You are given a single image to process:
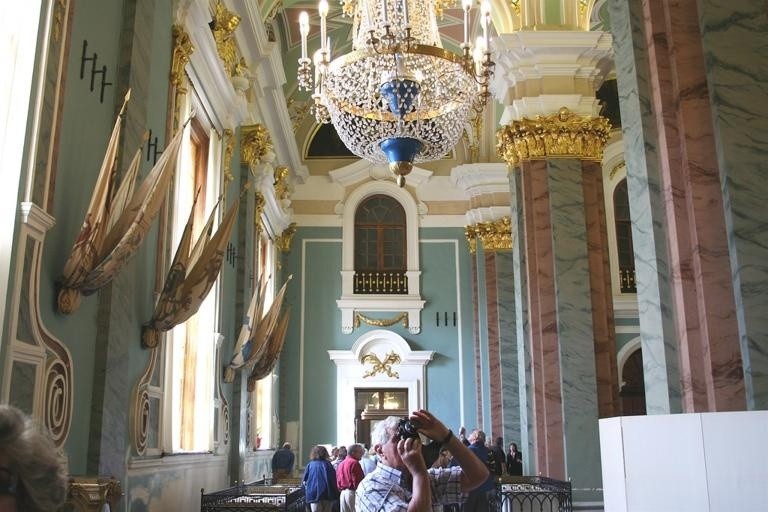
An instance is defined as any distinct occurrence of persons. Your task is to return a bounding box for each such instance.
[271,441,295,481]
[300,415,522,511]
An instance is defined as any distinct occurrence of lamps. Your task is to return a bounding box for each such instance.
[296,0,494,188]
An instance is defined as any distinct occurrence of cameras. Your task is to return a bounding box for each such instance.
[396,416,421,439]
